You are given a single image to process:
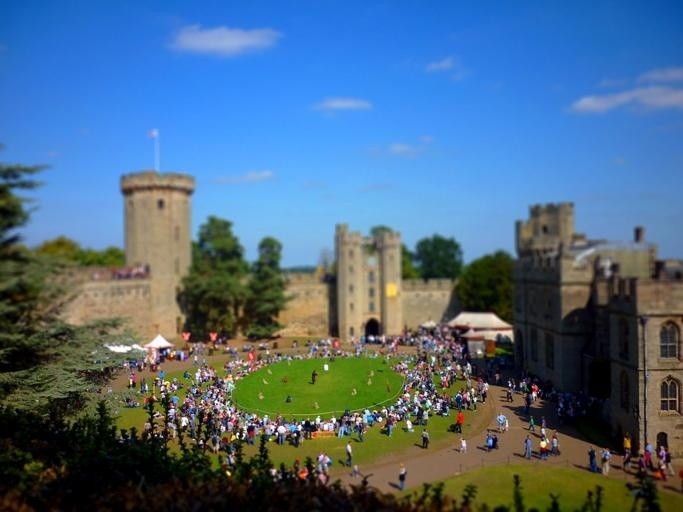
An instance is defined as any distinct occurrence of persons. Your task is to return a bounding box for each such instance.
[122,324,674,489]
